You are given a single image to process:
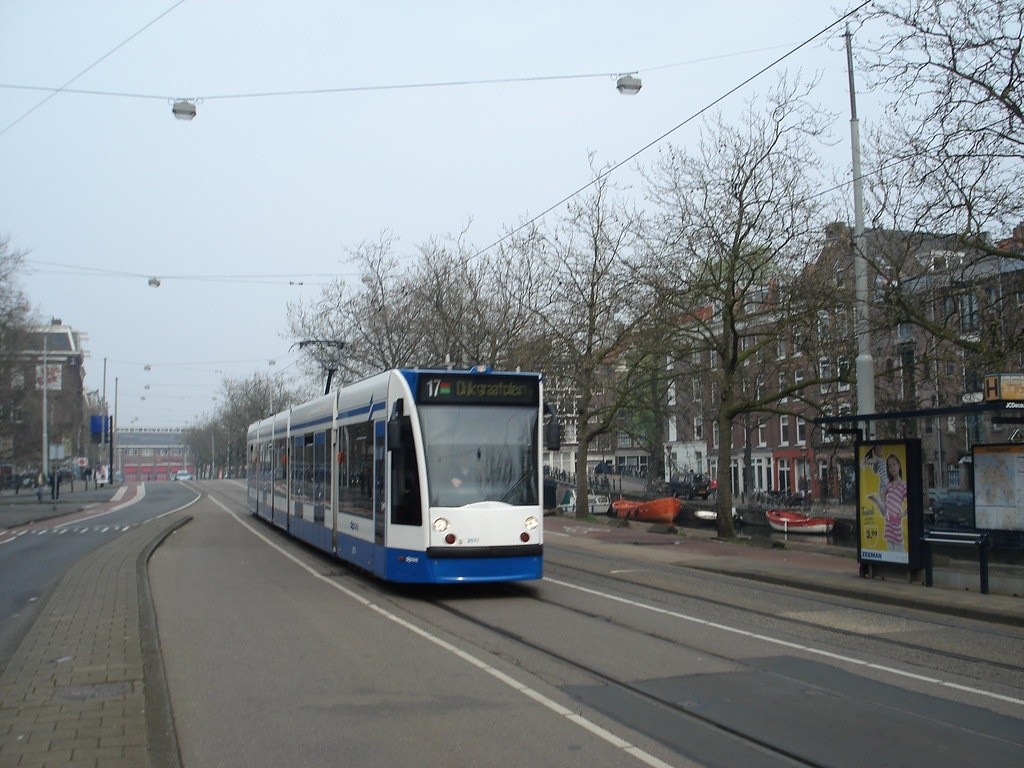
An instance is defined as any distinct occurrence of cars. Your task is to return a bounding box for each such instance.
[176,470,192,480]
[923,488,973,512]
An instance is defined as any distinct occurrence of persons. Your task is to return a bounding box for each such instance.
[867,453,907,552]
[863,446,888,497]
[49,471,61,503]
[84,466,92,479]
[444,458,481,489]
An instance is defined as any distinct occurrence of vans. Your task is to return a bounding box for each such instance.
[670,472,711,500]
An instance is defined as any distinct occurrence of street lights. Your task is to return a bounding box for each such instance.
[666,445,672,483]
[799,445,809,499]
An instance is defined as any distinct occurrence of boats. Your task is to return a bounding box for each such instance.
[766,509,834,534]
[557,488,684,523]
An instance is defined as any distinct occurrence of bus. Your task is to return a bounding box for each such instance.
[248,366,560,596]
